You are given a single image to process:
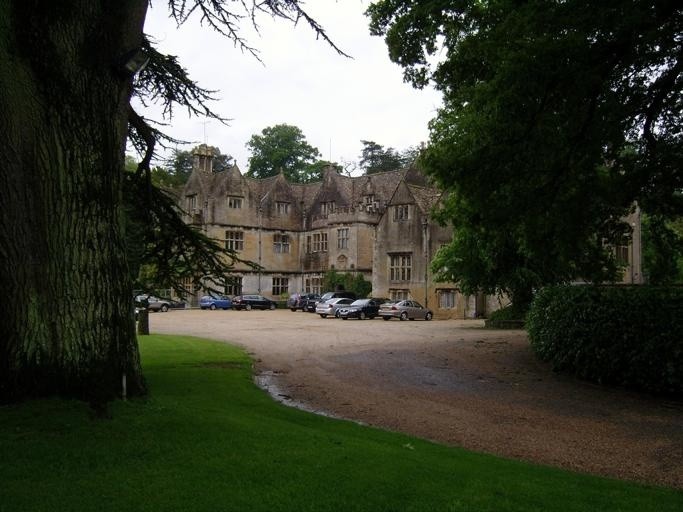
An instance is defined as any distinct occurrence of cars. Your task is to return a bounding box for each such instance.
[315,296,434,322]
[134,304,146,322]
[132,294,171,313]
[199,294,232,311]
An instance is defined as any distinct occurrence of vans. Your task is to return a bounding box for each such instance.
[230,294,278,310]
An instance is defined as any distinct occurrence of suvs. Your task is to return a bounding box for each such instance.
[304,290,358,313]
[285,292,320,313]
